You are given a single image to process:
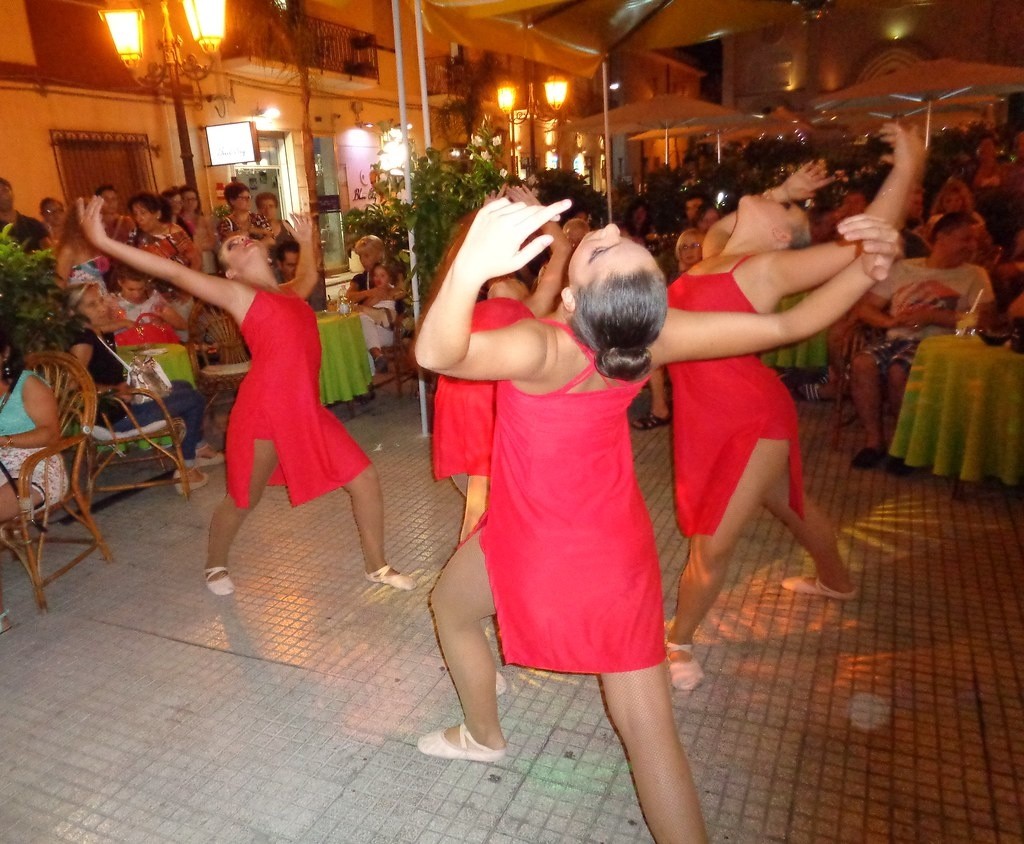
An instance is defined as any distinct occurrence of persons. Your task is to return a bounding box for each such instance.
[624,118,1024,476]
[65,278,224,493]
[0,333,69,633]
[72,194,416,595]
[419,180,570,695]
[1,172,399,460]
[416,196,904,844]
[652,120,926,687]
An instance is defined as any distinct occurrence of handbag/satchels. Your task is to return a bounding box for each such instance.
[112,312,181,347]
[122,356,172,405]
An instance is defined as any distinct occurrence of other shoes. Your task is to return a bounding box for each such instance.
[202,566,235,596]
[780,575,856,601]
[494,670,507,698]
[848,446,887,469]
[416,722,507,762]
[363,560,418,591]
[663,633,705,691]
[797,381,821,401]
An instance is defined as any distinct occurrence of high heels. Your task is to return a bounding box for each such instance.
[172,465,210,495]
[192,440,226,466]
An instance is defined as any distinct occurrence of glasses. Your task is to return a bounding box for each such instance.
[239,195,252,201]
[183,196,196,202]
[173,199,183,205]
[42,208,64,214]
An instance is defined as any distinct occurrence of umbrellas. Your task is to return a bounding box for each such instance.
[552,93,744,198]
[695,106,862,144]
[809,57,1024,151]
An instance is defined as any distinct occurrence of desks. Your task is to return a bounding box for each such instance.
[312,308,376,421]
[74,344,197,454]
[757,293,830,382]
[885,334,1024,503]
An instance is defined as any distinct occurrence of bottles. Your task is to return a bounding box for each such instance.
[338,285,350,316]
[1011,316,1024,353]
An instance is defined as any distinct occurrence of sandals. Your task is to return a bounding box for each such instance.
[0,607,12,635]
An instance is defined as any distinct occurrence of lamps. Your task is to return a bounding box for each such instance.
[352,102,375,130]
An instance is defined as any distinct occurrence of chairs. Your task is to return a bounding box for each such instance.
[0,351,115,615]
[60,358,192,506]
[359,302,418,401]
[187,300,252,454]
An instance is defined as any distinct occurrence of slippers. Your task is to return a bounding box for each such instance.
[631,409,672,429]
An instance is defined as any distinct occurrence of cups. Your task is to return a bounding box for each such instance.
[325,299,337,311]
[979,303,1010,345]
[134,322,150,350]
[955,308,979,340]
